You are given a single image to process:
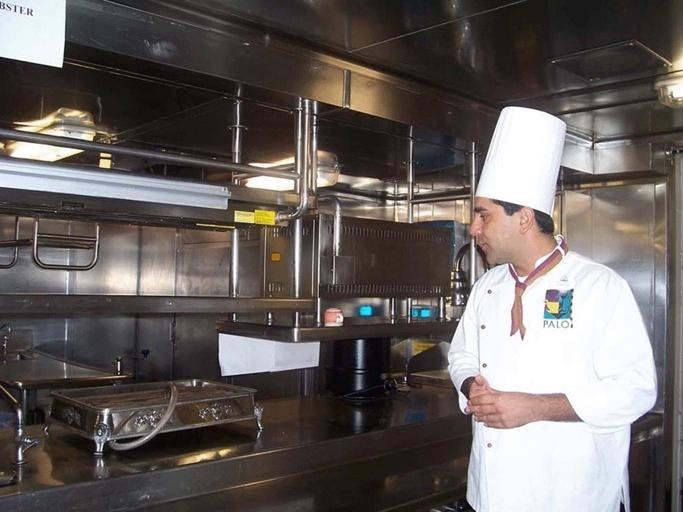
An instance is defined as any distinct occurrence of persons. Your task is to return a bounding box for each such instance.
[445,193,660,511]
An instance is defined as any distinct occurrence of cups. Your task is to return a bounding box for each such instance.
[323,307,344,327]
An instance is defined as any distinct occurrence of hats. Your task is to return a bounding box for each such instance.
[474,104,568,217]
